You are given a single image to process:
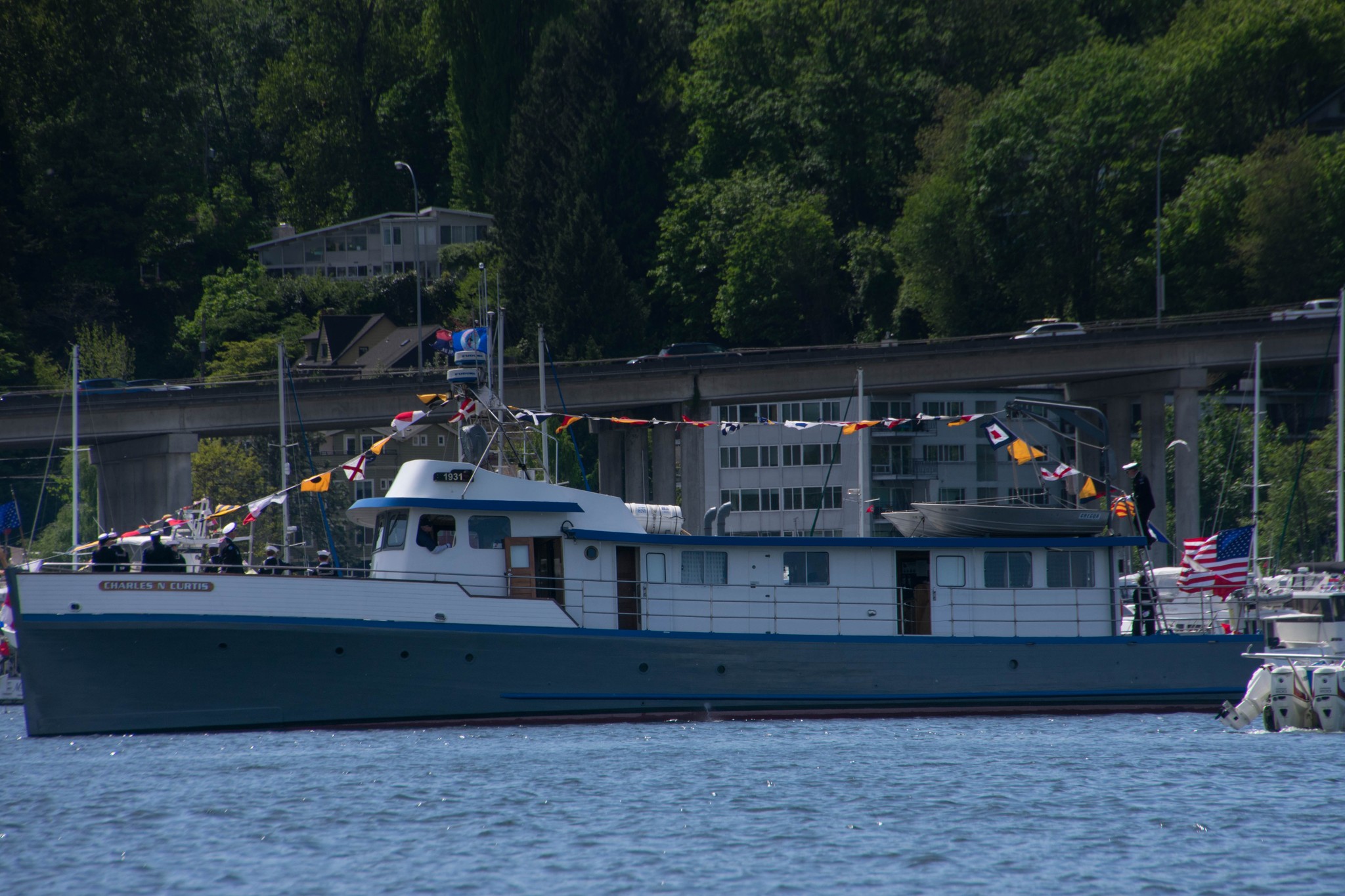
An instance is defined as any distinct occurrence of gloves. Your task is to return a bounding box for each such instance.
[1143,611,1149,618]
[446,543,451,548]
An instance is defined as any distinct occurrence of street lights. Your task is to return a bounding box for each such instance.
[1156,124,1183,331]
[393,160,424,384]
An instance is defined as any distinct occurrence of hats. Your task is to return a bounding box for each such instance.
[265,546,278,553]
[150,530,159,536]
[419,518,435,527]
[223,522,238,534]
[108,532,117,538]
[97,533,108,541]
[206,543,219,548]
[166,540,179,546]
[317,550,330,556]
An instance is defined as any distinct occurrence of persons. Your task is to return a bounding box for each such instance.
[258,546,314,575]
[1122,462,1156,549]
[311,550,339,577]
[140,531,187,574]
[204,543,222,573]
[1132,575,1158,636]
[219,522,244,574]
[416,518,451,553]
[92,533,131,574]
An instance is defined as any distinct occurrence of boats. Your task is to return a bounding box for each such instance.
[0,261,1271,739]
[1118,567,1345,735]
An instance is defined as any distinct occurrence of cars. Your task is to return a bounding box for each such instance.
[626,343,743,365]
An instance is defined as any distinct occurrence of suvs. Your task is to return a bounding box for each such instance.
[76,378,194,394]
[0,392,55,401]
[1008,323,1086,340]
[1271,300,1343,323]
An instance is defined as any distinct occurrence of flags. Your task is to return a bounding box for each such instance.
[370,393,457,458]
[1176,524,1256,602]
[0,500,21,534]
[980,416,1080,481]
[429,327,490,357]
[507,405,985,436]
[448,396,485,424]
[1079,476,1170,544]
[341,452,366,481]
[0,559,44,664]
[301,470,332,492]
[65,490,287,554]
